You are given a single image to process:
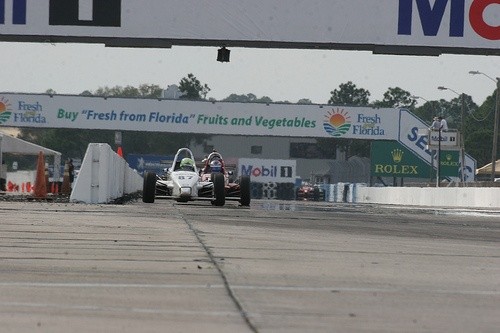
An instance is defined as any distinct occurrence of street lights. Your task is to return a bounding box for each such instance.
[409,95,433,125]
[469,70,500,183]
[437,86,465,187]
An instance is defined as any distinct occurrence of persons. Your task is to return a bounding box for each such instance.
[430,117,448,131]
[175,158,194,171]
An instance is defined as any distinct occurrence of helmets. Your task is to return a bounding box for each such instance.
[210,160,221,167]
[180,158,194,168]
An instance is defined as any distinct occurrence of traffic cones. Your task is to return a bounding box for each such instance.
[44,160,50,193]
[58,160,71,195]
[117,146,123,158]
[24,150,53,200]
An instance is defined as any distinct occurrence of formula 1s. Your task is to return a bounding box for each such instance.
[143,148,250,207]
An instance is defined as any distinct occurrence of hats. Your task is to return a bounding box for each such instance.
[433,117,439,120]
